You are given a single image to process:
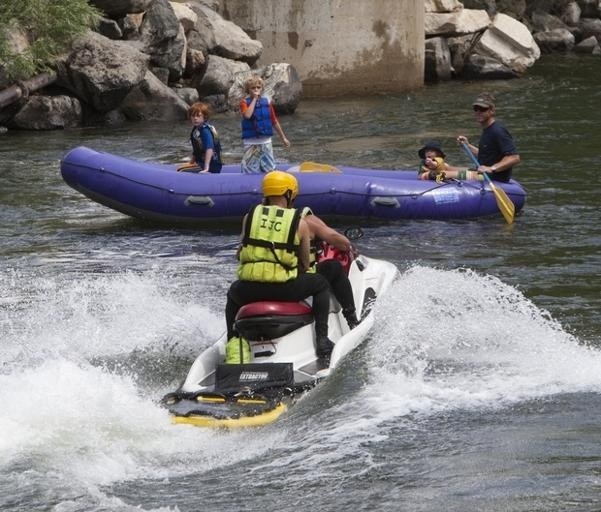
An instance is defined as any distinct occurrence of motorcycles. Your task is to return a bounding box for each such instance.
[150,224,405,432]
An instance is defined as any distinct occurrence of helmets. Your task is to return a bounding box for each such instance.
[262,170,298,198]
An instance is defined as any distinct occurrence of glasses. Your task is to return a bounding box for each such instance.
[473,105,488,112]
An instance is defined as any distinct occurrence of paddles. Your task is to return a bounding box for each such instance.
[460,139,515,225]
[299,162,342,173]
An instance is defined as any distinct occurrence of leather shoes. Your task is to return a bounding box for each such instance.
[316,336,336,355]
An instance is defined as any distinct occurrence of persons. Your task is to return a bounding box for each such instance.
[416,138,452,180]
[225,170,339,361]
[289,173,378,332]
[437,90,523,184]
[176,99,224,177]
[238,73,293,174]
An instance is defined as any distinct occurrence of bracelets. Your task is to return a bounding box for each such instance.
[491,166,498,174]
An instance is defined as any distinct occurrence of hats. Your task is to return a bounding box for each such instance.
[472,92,496,109]
[418,140,446,158]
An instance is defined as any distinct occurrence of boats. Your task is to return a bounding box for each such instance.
[56,143,529,231]
